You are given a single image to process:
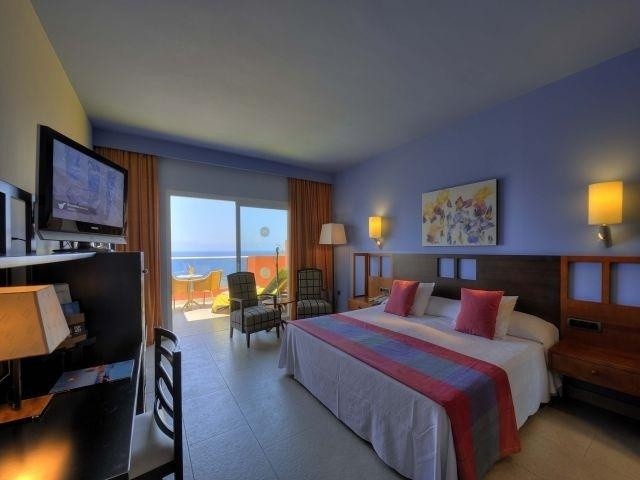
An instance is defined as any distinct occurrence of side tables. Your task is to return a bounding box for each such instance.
[262,297,297,337]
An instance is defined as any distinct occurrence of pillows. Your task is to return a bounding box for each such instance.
[454,287,503,341]
[491,295,522,339]
[383,279,418,318]
[408,281,437,318]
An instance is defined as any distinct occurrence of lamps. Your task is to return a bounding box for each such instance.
[0,285,71,424]
[368,216,384,250]
[317,222,348,313]
[586,180,623,248]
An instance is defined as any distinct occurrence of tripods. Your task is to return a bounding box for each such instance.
[266,261,288,333]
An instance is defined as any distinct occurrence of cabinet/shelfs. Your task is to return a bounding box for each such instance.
[34,250,145,415]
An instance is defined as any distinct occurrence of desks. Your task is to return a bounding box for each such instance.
[1,343,144,480]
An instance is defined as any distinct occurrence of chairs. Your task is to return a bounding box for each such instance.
[171,265,291,313]
[296,267,334,319]
[107,325,183,479]
[226,271,281,347]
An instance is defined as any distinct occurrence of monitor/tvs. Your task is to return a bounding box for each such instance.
[35,123,127,254]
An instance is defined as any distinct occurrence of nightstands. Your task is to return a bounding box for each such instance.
[347,296,382,312]
[547,338,640,420]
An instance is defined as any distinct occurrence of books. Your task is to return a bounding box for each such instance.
[48,358,135,394]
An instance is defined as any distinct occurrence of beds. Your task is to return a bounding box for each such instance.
[283,295,558,479]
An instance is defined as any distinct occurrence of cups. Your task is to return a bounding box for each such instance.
[100,243,111,250]
[36,234,53,255]
